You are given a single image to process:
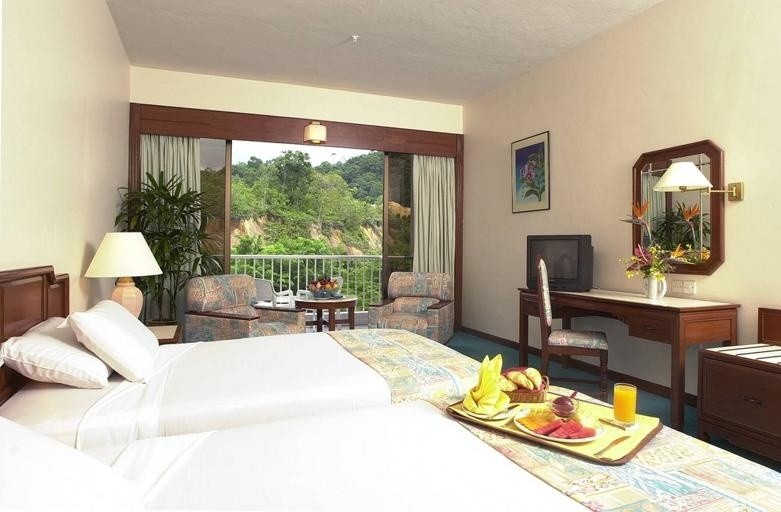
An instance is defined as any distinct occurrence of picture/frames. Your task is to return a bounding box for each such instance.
[511,131,550,213]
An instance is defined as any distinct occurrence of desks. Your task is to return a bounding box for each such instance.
[518,288,740,433]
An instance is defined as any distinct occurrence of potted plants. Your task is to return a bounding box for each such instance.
[114,170,224,343]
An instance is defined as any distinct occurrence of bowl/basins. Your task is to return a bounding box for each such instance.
[307,277,343,298]
[550,396,579,418]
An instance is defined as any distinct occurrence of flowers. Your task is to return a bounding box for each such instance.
[618,197,685,278]
[308,277,339,292]
[679,202,710,265]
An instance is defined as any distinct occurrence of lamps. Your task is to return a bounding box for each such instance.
[83,232,163,319]
[653,162,744,202]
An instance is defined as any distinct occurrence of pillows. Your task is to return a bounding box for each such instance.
[57,297,160,385]
[1,316,115,390]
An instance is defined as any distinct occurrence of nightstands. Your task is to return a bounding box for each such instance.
[147,325,179,343]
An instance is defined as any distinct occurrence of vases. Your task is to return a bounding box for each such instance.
[641,277,667,300]
[314,291,332,298]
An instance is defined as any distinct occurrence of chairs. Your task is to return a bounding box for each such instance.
[368,271,454,344]
[535,259,608,400]
[184,274,306,343]
[254,277,293,308]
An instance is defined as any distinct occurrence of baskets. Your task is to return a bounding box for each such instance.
[501,366,549,403]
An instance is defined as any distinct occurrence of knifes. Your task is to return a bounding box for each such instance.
[594,436,634,458]
[487,405,527,424]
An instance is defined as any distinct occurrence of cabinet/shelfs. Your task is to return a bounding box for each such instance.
[697,307,781,448]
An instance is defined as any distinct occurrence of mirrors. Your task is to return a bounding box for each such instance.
[632,139,725,276]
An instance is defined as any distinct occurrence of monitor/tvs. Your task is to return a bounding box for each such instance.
[527,235,592,292]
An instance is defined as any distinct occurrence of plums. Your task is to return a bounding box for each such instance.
[553,396,573,418]
[326,277,330,282]
[310,280,317,284]
[321,279,326,285]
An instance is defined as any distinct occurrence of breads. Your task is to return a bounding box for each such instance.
[500,367,542,392]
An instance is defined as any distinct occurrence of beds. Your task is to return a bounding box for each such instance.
[0,264,481,448]
[0,384,781,511]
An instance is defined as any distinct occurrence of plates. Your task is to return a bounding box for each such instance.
[461,401,516,422]
[514,406,607,444]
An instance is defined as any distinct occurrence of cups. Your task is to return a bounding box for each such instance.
[612,381,638,427]
